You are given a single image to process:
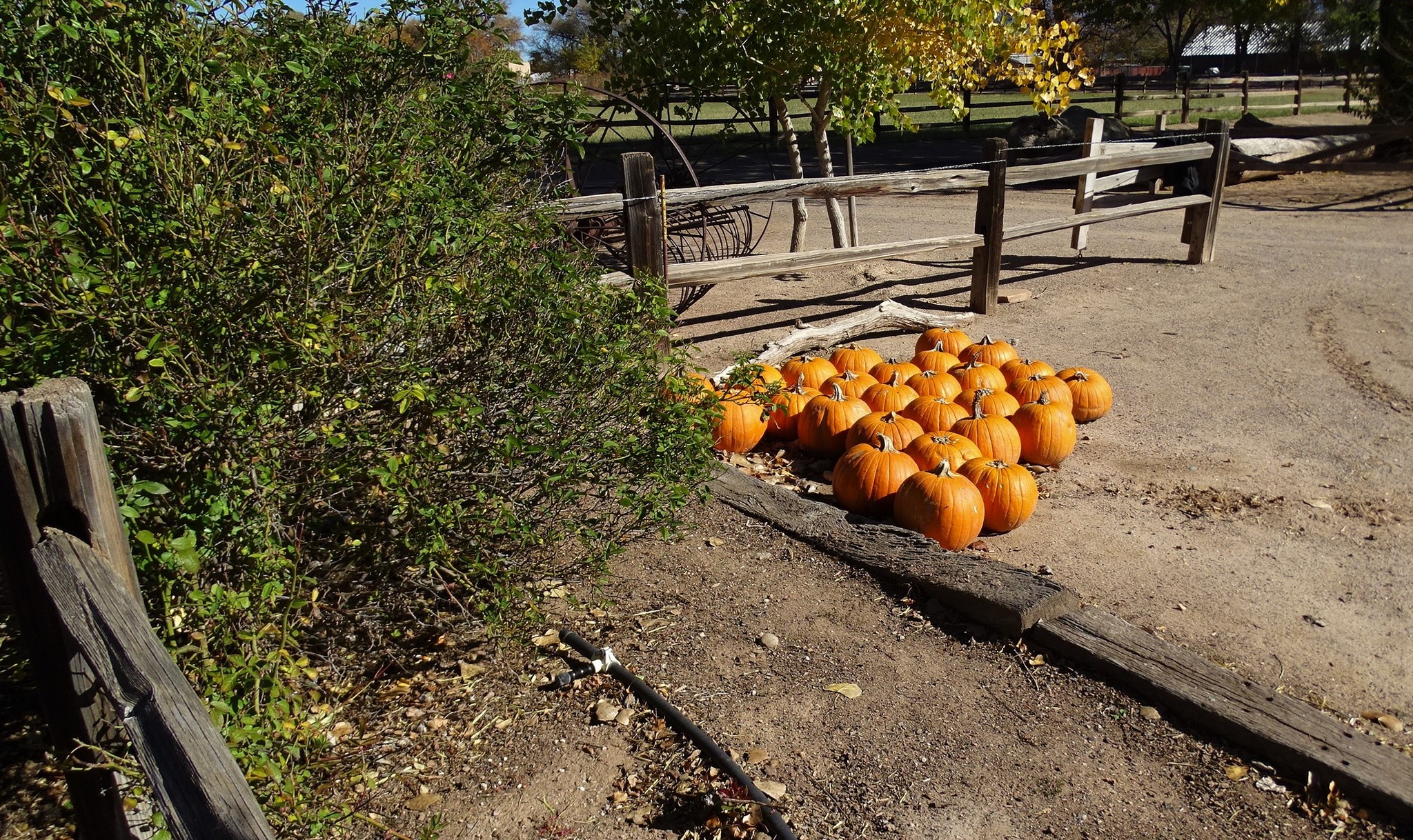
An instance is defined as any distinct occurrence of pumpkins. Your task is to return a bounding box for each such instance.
[831,432,920,521]
[722,362,786,396]
[797,383,873,459]
[892,459,985,552]
[956,457,1038,532]
[695,390,770,454]
[845,411,925,451]
[765,372,825,440]
[780,355,838,391]
[660,372,713,411]
[905,431,983,472]
[818,369,879,399]
[828,342,884,374]
[860,328,1112,466]
